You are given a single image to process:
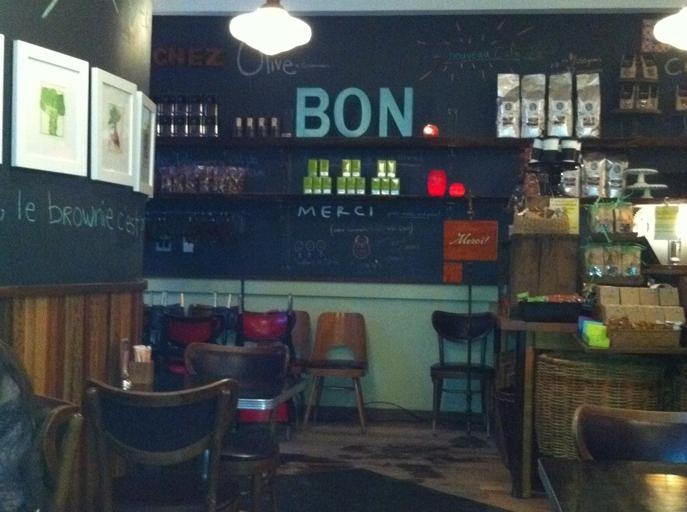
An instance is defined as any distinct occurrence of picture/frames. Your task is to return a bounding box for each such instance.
[139,91,158,195]
[11,42,88,176]
[90,67,139,190]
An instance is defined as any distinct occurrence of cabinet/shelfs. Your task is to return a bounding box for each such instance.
[155,133,515,207]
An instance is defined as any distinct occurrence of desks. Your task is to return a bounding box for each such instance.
[120,371,307,411]
[491,319,687,499]
[538,460,687,512]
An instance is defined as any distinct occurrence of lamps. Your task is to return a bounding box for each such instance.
[231,1,311,57]
[653,1,687,51]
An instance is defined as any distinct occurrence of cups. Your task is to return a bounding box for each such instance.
[530,138,542,163]
[542,139,558,164]
[131,362,155,386]
[669,240,681,264]
[577,143,583,162]
[354,236,370,262]
[562,140,577,163]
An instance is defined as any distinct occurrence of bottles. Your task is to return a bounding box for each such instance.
[209,101,222,137]
[270,118,279,137]
[450,183,465,197]
[181,97,194,137]
[196,97,208,135]
[164,96,179,137]
[153,96,164,137]
[157,163,246,195]
[234,118,242,138]
[258,118,265,138]
[427,170,448,198]
[246,117,253,137]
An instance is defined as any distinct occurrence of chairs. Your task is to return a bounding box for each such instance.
[31,394,85,512]
[305,312,368,432]
[155,315,218,379]
[573,405,687,462]
[235,310,295,429]
[291,310,311,372]
[84,378,249,511]
[186,344,290,511]
[431,311,494,426]
